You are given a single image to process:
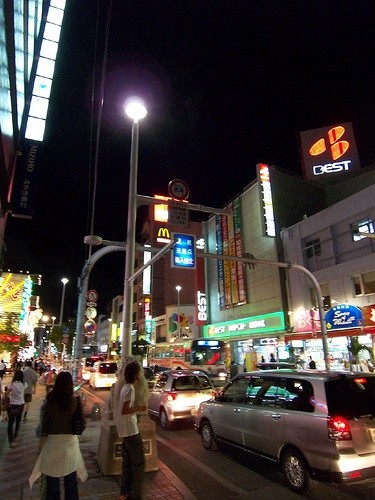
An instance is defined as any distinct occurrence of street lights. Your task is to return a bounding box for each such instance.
[58,277,70,328]
[96,95,160,476]
[175,284,182,339]
[137,302,142,347]
[107,318,112,362]
[50,315,57,330]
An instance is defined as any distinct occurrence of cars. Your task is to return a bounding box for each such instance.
[243,362,297,372]
[143,370,219,430]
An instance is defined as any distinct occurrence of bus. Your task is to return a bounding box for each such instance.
[142,337,231,388]
[82,344,99,359]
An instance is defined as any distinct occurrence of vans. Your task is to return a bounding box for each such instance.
[81,357,104,381]
[88,361,118,391]
[194,371,375,496]
